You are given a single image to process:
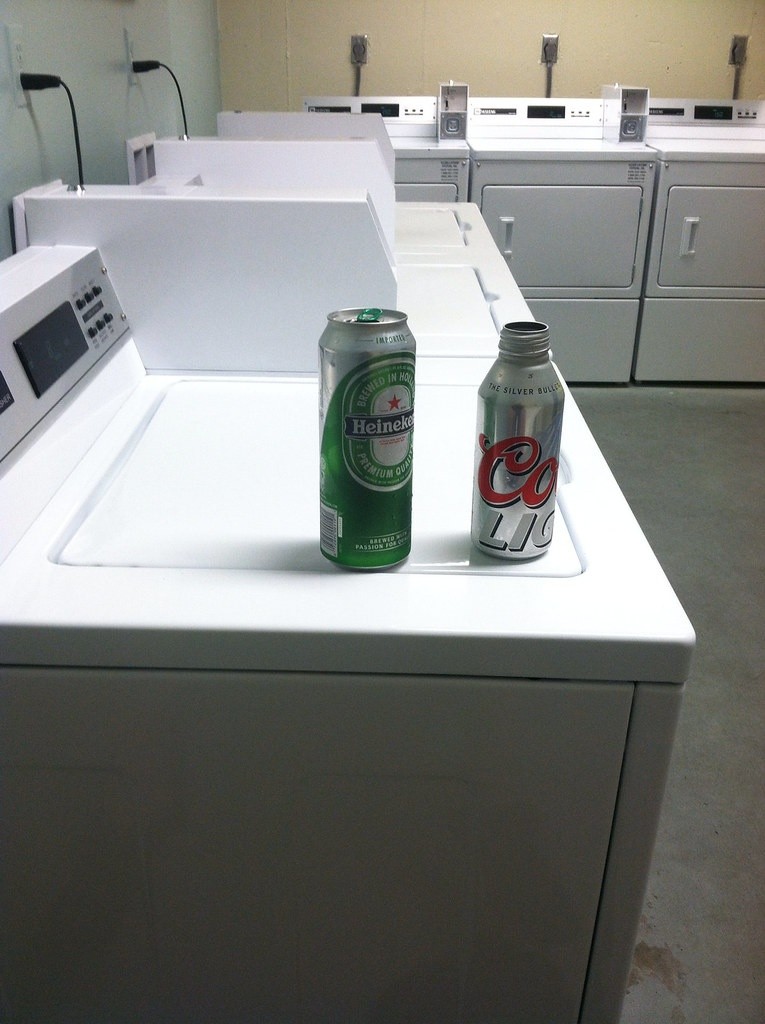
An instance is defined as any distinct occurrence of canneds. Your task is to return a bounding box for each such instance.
[319,310,566,571]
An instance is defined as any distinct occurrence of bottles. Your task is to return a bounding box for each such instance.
[473,322,567,563]
